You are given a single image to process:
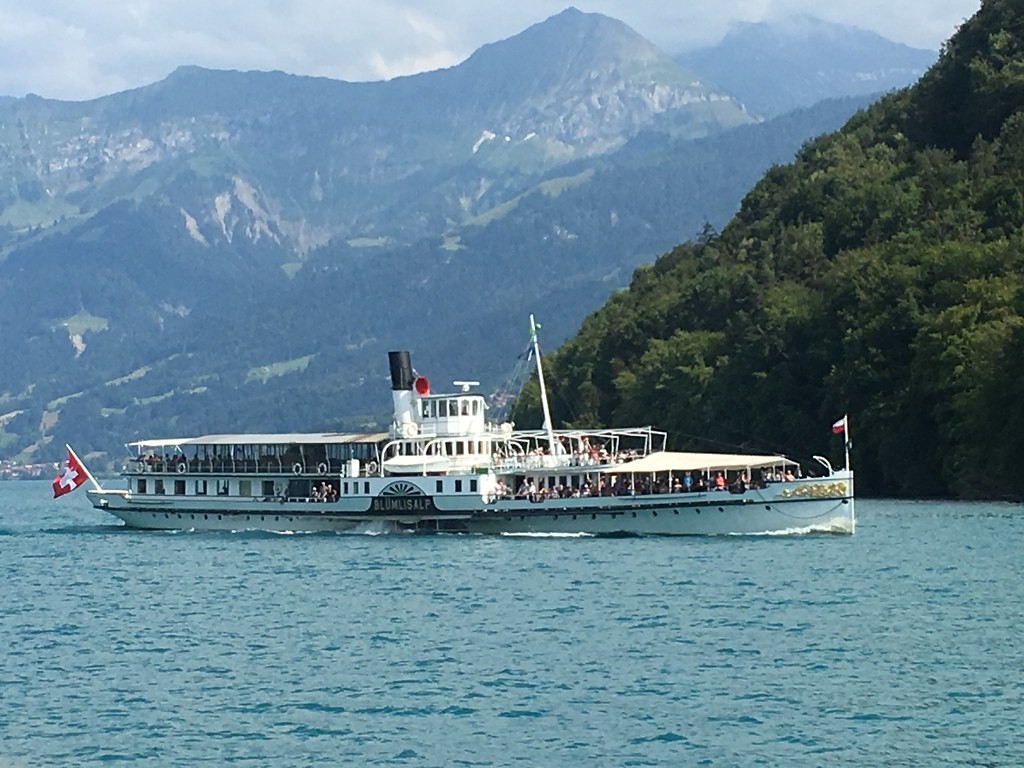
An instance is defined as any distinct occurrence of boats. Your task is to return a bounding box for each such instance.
[52,317,855,535]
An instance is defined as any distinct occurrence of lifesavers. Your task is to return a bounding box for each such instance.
[292,462,302,475]
[367,461,378,474]
[178,463,186,474]
[318,463,327,476]
[135,461,145,473]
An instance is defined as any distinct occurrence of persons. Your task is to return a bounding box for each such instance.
[309,481,337,504]
[488,441,797,500]
[139,451,377,471]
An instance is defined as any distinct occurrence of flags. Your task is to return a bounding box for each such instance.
[519,348,541,362]
[833,416,846,433]
[52,451,90,498]
